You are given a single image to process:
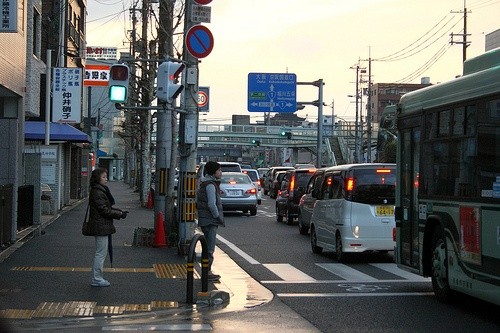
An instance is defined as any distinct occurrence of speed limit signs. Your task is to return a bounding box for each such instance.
[198,86,210,112]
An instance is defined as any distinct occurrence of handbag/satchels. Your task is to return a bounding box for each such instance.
[82,221,90,236]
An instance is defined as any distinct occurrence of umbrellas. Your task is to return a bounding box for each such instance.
[108,235,112,268]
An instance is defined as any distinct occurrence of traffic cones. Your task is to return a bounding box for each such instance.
[154,211,168,247]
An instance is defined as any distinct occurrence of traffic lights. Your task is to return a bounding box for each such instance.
[108,63,130,104]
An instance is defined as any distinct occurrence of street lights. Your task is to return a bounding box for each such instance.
[347,94,364,161]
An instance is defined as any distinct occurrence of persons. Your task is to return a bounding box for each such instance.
[197,161,225,280]
[89,167,129,287]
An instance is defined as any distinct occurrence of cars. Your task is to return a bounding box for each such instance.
[196,164,213,192]
[174,164,199,188]
[219,172,258,216]
[240,168,262,205]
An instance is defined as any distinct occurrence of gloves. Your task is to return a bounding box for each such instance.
[121,212,127,219]
[122,210,128,214]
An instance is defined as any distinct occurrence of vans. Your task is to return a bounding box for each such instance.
[275,169,317,225]
[297,166,329,236]
[308,162,398,258]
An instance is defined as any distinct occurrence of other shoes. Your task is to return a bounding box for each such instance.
[207,273,221,280]
[90,278,110,287]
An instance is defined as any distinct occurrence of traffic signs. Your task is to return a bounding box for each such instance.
[247,72,296,112]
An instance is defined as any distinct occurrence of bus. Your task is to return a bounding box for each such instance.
[378,47,500,305]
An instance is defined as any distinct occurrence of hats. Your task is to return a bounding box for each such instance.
[203,161,220,177]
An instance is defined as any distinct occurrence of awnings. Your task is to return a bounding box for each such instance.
[97,150,107,156]
[24,120,92,143]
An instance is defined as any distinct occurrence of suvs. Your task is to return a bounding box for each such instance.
[241,165,251,169]
[263,164,316,198]
[257,168,269,177]
[217,161,241,172]
